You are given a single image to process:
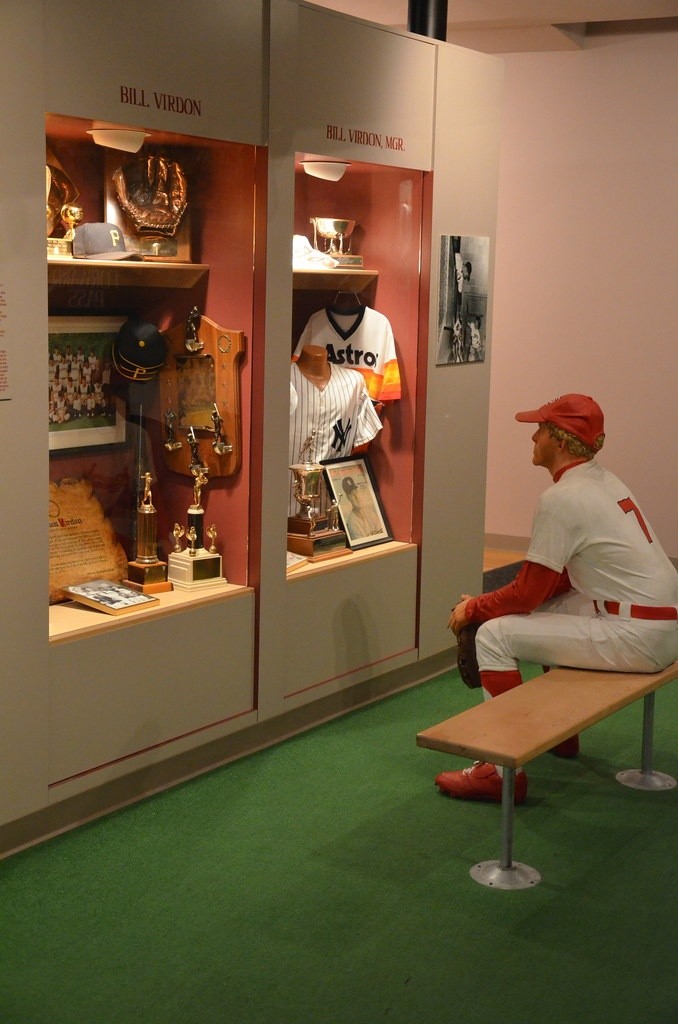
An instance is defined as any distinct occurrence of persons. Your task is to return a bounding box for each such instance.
[341,476,384,540]
[48,345,114,423]
[288,345,383,516]
[455,252,472,316]
[452,317,481,363]
[186,434,200,465]
[435,393,678,800]
[211,410,225,445]
[81,584,149,610]
[164,409,177,443]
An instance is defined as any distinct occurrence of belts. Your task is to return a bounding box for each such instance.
[594,598,678,620]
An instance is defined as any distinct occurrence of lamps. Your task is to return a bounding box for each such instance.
[86,129,151,154]
[299,160,351,182]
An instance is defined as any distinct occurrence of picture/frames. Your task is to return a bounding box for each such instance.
[46,312,133,452]
[318,454,398,551]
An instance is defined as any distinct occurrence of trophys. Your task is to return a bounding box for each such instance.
[123,473,173,594]
[309,217,364,269]
[287,430,346,556]
[167,464,227,592]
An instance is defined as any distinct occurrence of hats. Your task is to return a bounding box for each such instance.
[73,222,145,260]
[515,393,605,454]
[466,262,472,281]
[341,477,358,493]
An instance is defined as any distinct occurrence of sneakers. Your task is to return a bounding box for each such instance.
[549,734,580,758]
[435,758,529,804]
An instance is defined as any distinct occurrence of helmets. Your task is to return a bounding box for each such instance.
[111,321,165,383]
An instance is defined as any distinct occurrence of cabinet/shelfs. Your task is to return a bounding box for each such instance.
[0,0,504,863]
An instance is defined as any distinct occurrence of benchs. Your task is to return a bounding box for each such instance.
[411,663,678,896]
